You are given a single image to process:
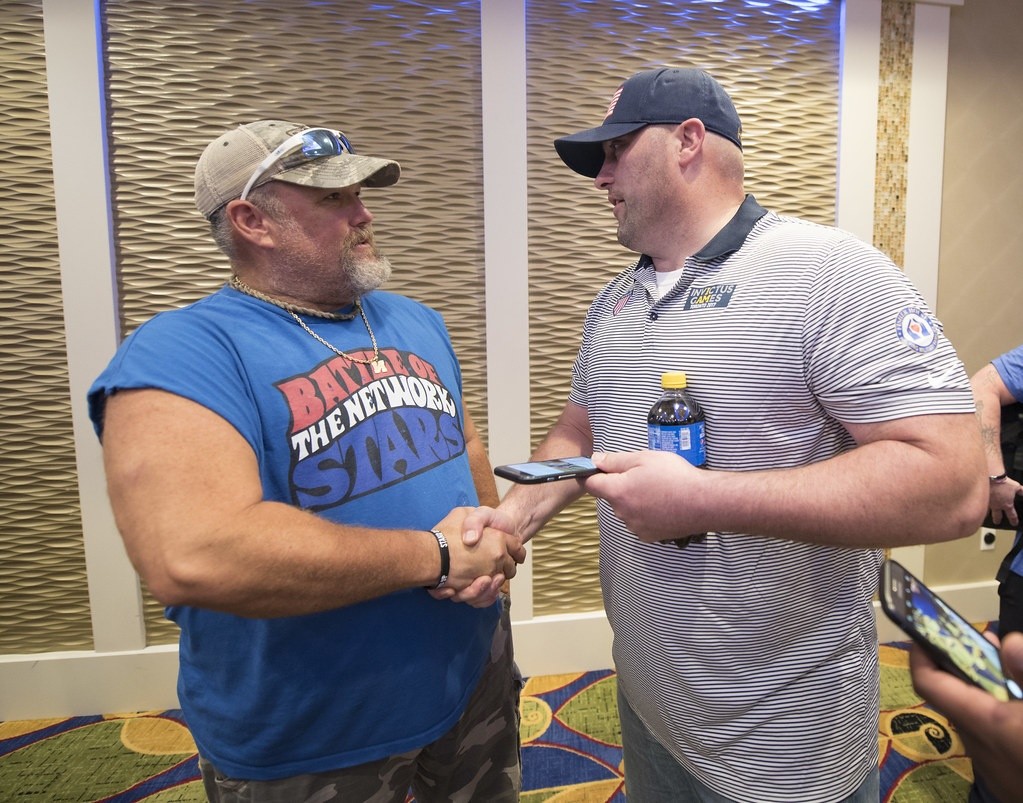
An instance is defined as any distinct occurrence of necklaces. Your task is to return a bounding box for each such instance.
[228,274,378,363]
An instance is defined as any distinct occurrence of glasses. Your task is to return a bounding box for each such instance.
[241,126,351,202]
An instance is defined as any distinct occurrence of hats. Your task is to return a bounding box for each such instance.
[554,67,742,179]
[192,120,401,219]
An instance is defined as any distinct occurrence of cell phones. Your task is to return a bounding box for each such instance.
[880,558,1022,703]
[493,457,601,486]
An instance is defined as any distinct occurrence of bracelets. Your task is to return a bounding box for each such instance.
[989,472,1007,481]
[423,529,450,591]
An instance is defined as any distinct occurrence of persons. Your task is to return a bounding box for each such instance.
[968,343,1023,640]
[87,119,527,803]
[430,67,990,803]
[909,631,1023,803]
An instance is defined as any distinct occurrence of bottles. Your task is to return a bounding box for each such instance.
[648,371,706,548]
